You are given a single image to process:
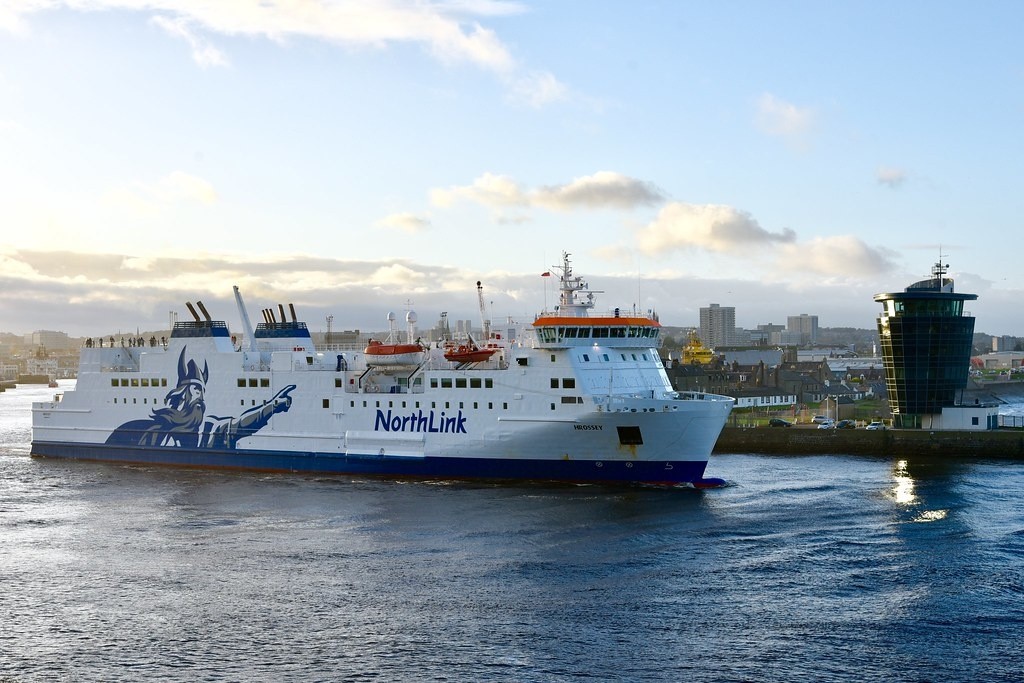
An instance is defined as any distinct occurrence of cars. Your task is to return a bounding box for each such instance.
[865,421,886,432]
[769,418,792,428]
[817,421,835,430]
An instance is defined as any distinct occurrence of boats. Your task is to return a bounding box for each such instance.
[48,377,59,388]
[443,332,497,363]
[29,247,739,493]
[363,337,425,366]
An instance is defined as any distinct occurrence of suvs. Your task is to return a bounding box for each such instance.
[811,415,835,424]
[836,419,857,430]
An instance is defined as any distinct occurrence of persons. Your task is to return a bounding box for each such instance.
[128,337,144,347]
[161,336,165,346]
[415,337,420,344]
[86,338,95,348]
[232,336,236,344]
[121,337,124,347]
[458,344,478,351]
[110,337,114,346]
[99,338,103,347]
[149,336,155,347]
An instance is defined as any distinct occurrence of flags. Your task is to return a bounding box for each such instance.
[541,272,550,276]
